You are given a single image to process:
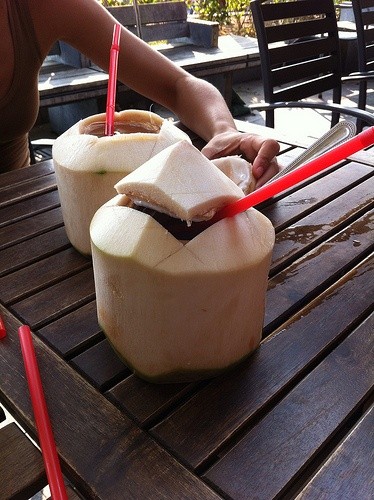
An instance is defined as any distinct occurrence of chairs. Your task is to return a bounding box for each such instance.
[351,0,374,133]
[251,0,373,134]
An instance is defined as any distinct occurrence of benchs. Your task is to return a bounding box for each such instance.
[39,3,263,110]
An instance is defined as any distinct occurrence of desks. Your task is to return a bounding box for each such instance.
[1,136,374,500]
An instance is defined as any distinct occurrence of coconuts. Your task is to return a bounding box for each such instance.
[89,139,276,384]
[211,155,257,197]
[52,108,194,254]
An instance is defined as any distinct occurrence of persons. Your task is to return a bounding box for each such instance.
[1,1,279,190]
